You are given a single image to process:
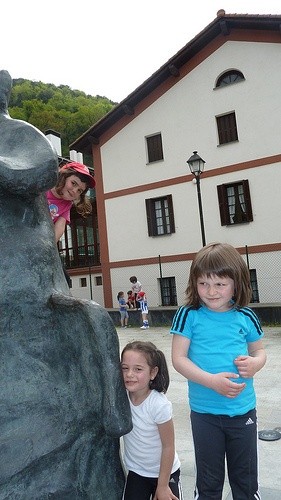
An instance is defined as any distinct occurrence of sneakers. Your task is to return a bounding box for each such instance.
[140,324,146,328]
[147,325,149,329]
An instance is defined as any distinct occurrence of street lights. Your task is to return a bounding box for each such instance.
[186,151,206,248]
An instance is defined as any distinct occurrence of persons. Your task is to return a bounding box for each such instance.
[169,241,267,500]
[121,341,183,500]
[136,291,150,329]
[45,162,96,242]
[127,276,144,310]
[117,292,131,329]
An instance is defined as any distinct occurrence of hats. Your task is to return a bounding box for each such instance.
[137,292,145,296]
[58,162,96,189]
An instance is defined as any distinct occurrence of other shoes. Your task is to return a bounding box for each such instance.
[120,326,125,329]
[125,325,131,328]
